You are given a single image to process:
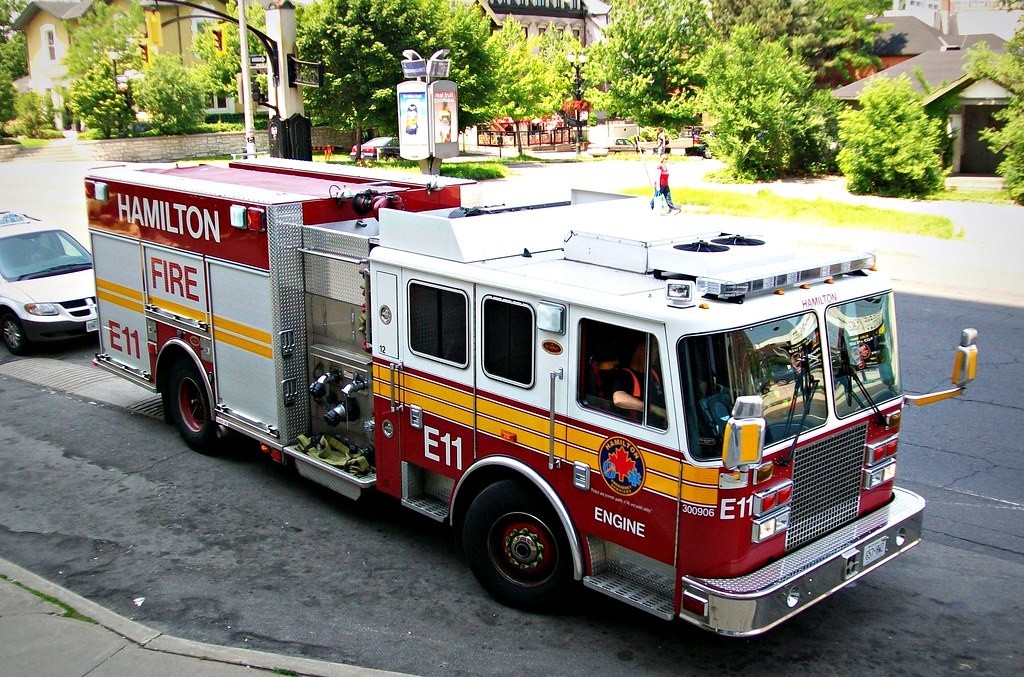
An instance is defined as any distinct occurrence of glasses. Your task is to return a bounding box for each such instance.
[664,159,667,160]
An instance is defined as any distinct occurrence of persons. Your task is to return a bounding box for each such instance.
[610,339,664,417]
[656,127,665,155]
[650,154,676,210]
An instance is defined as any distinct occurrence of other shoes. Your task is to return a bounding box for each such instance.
[669,207,679,212]
[648,207,655,211]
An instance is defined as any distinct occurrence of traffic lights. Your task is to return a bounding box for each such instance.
[211,27,222,51]
[138,42,148,63]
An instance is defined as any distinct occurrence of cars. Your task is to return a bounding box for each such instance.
[0,210,99,355]
[587,138,646,158]
[350,137,400,161]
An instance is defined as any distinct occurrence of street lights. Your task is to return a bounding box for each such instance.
[139,0,286,158]
[108,51,119,89]
[568,54,587,154]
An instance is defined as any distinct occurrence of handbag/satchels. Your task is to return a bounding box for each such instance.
[654,191,669,217]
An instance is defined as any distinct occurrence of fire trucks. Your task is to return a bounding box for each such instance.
[83,156,979,640]
